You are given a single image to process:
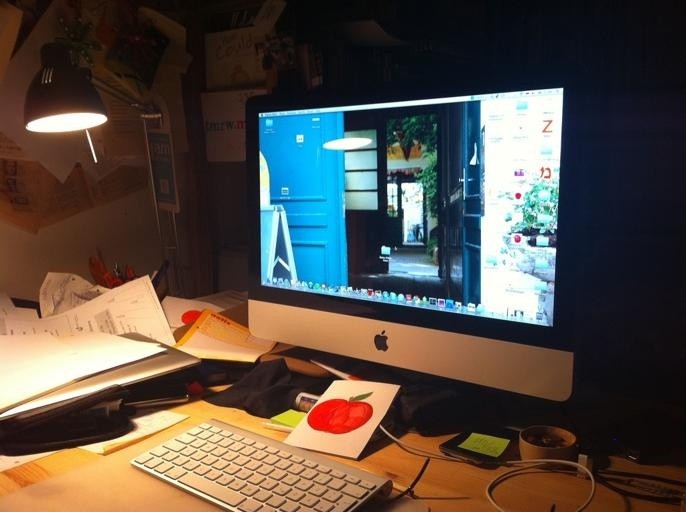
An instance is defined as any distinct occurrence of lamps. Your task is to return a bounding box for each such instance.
[22,38,189,301]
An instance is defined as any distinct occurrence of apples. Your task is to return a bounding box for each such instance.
[307,392,372,434]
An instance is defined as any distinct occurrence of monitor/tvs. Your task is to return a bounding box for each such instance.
[246,78,576,405]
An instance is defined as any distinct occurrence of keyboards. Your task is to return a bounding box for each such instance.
[129,417,394,512]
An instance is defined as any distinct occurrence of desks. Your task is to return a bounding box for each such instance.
[0,294,686,511]
